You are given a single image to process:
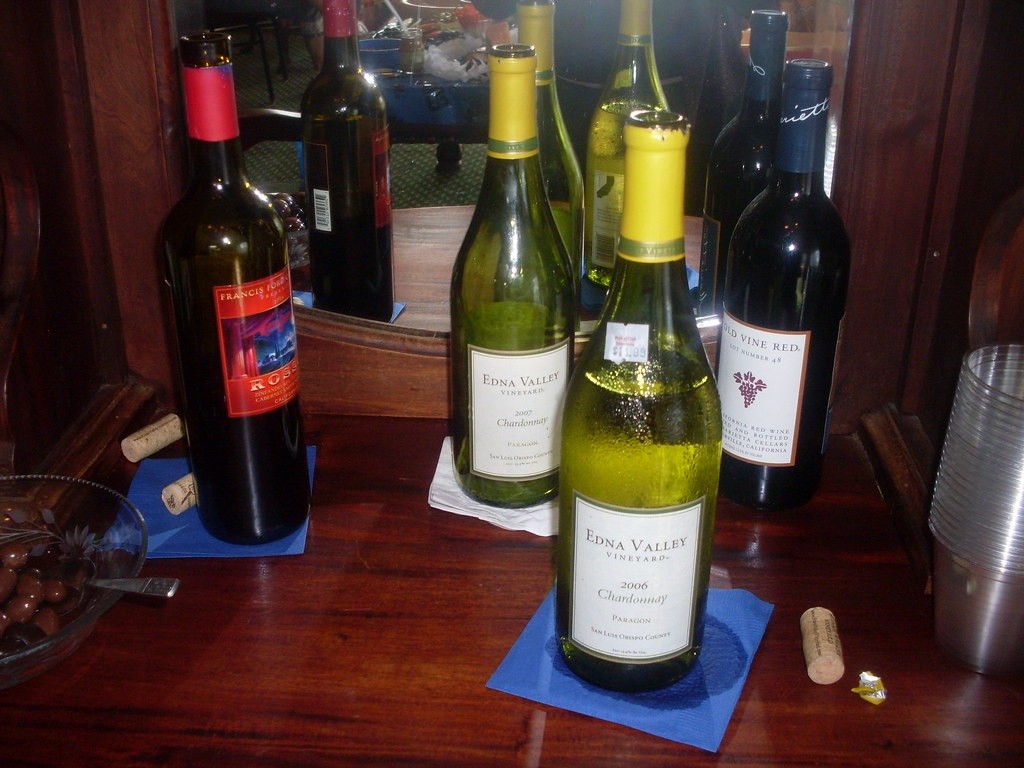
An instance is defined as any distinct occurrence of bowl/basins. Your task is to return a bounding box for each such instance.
[356,37,402,71]
[264,192,311,292]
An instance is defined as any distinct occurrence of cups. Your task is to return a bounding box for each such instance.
[928,343,1024,670]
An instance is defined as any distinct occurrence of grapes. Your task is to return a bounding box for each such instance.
[264,191,312,292]
[0,541,65,663]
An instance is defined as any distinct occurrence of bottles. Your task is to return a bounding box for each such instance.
[450,43,578,509]
[509,0,586,317]
[160,30,311,547]
[584,0,672,289]
[553,107,723,692]
[301,1,398,323]
[718,60,848,512]
[399,28,425,74]
[698,11,789,314]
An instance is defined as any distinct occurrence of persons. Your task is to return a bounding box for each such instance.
[456,0,770,217]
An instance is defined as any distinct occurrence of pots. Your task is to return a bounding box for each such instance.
[0,472,149,693]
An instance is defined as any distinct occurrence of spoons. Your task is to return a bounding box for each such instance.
[63,558,180,596]
